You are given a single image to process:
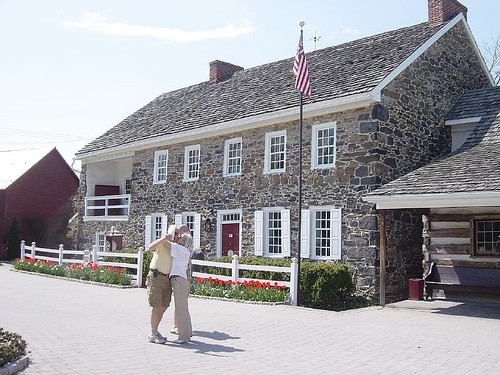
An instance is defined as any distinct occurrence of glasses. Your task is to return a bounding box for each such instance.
[175,232,179,235]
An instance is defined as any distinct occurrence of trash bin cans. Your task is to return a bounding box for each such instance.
[408,279,424,300]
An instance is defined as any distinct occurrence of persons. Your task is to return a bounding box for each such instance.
[146,224,194,344]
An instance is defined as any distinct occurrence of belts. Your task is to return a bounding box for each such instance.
[150,268,169,277]
[170,275,181,279]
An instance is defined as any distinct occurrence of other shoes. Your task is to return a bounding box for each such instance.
[170,329,178,334]
[173,339,190,344]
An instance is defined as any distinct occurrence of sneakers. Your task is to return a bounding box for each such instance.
[148,331,167,340]
[149,336,166,344]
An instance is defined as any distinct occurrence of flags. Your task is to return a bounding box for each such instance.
[292,31,313,102]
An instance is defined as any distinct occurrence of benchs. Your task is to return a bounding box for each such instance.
[423,262,500,301]
